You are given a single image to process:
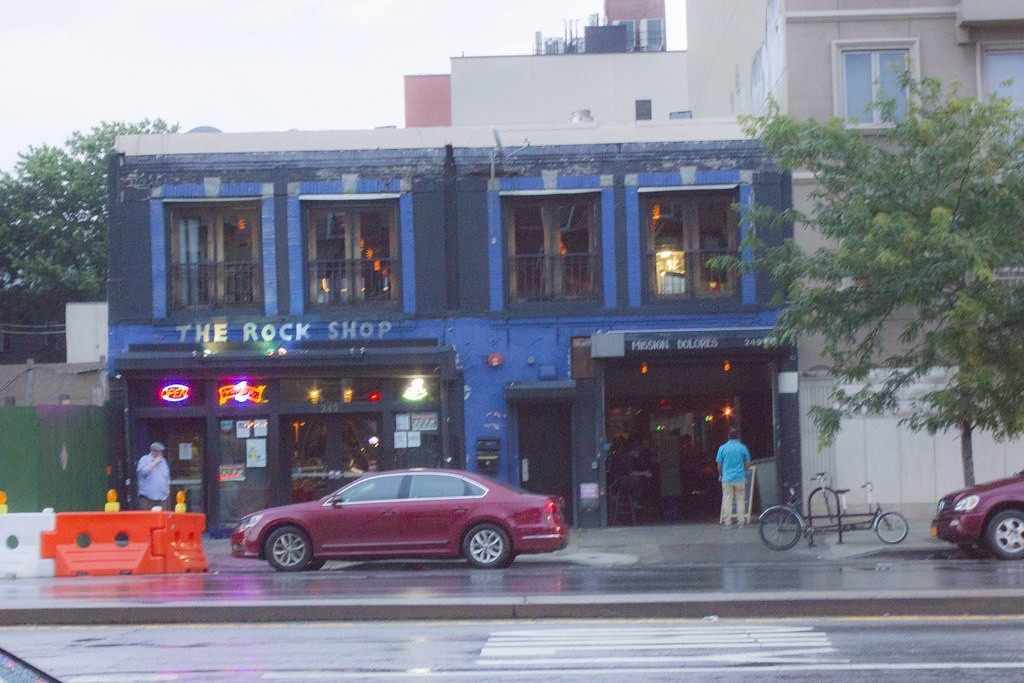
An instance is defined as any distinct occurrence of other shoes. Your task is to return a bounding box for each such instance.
[720,523,733,529]
[737,522,745,529]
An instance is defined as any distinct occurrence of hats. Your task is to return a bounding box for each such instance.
[150,442,165,451]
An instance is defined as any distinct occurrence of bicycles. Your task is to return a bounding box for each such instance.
[757,473,909,550]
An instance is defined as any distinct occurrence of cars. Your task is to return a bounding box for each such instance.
[228,469,571,570]
[930,468,1024,558]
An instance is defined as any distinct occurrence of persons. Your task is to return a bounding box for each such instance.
[612,425,718,522]
[136,442,170,512]
[716,428,751,530]
